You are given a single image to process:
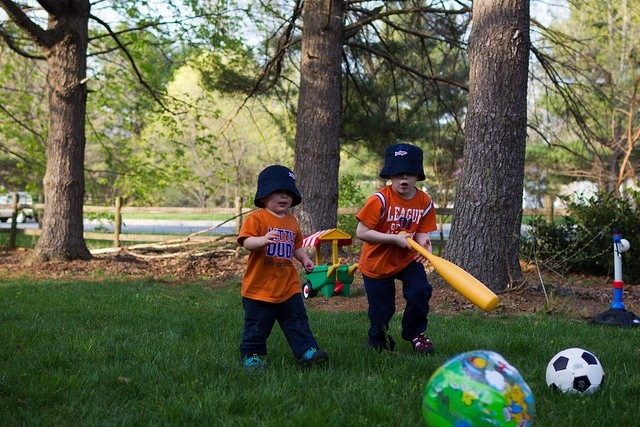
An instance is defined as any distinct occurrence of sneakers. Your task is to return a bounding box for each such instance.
[295,347,329,372]
[400,329,435,357]
[241,354,268,369]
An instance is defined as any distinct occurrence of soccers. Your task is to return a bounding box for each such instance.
[546,347,604,396]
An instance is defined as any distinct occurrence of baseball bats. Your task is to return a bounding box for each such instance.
[398,231,498,312]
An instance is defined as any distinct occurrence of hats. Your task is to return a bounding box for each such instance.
[379,143,426,181]
[254,166,302,208]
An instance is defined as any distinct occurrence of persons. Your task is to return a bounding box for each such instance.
[236,165,329,367]
[356,143,435,354]
